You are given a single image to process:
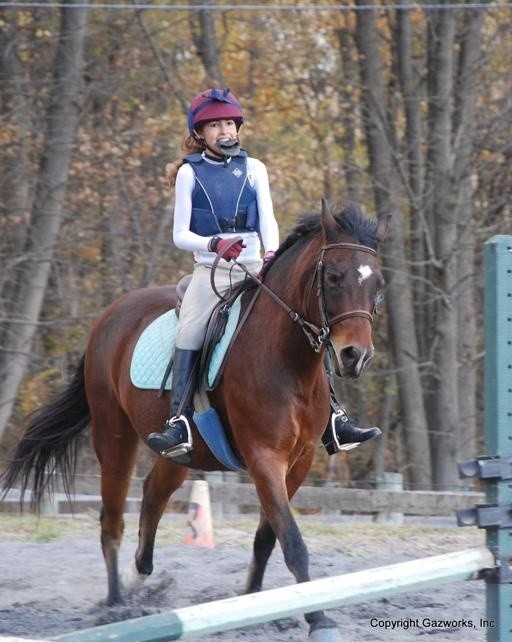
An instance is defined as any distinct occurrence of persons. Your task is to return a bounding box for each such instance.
[147,88,382,465]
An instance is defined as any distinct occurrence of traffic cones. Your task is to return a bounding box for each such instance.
[181,480,215,548]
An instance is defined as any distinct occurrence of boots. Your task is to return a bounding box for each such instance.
[147,345,202,464]
[321,404,382,456]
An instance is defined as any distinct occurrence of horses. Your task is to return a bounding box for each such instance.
[0,195,393,642]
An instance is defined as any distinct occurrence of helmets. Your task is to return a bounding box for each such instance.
[188,89,243,135]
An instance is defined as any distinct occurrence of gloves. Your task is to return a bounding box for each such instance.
[263,251,277,265]
[208,237,247,262]
